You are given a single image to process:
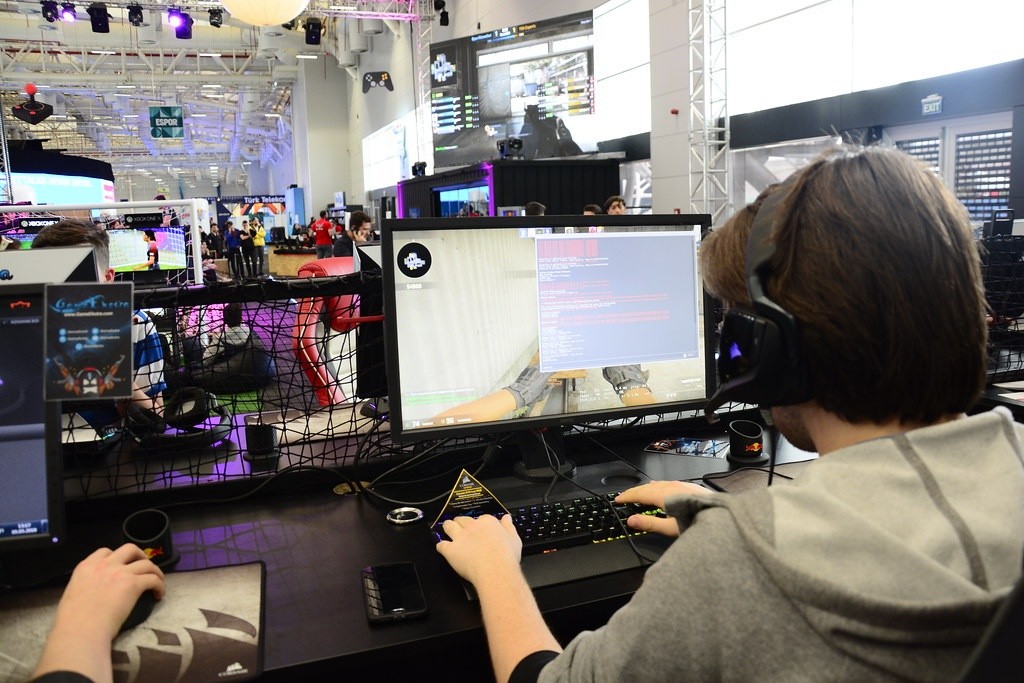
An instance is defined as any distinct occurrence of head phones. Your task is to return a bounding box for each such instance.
[717,156,833,410]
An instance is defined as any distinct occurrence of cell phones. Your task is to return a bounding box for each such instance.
[361,560,429,625]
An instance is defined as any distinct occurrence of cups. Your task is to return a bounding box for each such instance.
[245,423,278,456]
[728,420,763,459]
[123,509,173,565]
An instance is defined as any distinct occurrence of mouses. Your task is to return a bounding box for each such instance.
[120,586,156,631]
[360,396,389,419]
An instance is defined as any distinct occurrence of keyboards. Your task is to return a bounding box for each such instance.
[427,488,679,604]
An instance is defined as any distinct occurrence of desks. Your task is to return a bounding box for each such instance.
[0,369,1024,683]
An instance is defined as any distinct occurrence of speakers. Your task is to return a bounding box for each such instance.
[306,17,321,46]
[175,14,192,39]
[90,3,110,33]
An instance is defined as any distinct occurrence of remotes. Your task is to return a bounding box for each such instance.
[379,213,725,511]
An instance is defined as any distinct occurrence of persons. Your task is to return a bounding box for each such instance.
[31,218,167,434]
[584,197,625,215]
[185,218,266,280]
[202,305,254,374]
[525,65,544,95]
[28,542,165,683]
[134,230,161,270]
[310,211,341,259]
[436,145,1023,683]
[292,223,303,235]
[324,212,372,257]
[95,219,124,228]
[154,195,180,227]
[525,202,545,215]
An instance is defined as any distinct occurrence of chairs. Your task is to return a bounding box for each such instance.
[292,256,361,406]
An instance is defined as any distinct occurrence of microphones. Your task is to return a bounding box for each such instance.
[705,373,768,426]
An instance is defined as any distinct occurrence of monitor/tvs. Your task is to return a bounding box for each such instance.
[0,244,111,590]
[104,226,187,289]
[356,242,388,400]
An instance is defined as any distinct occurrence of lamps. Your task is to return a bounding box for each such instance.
[496,138,523,158]
[26,0,450,178]
